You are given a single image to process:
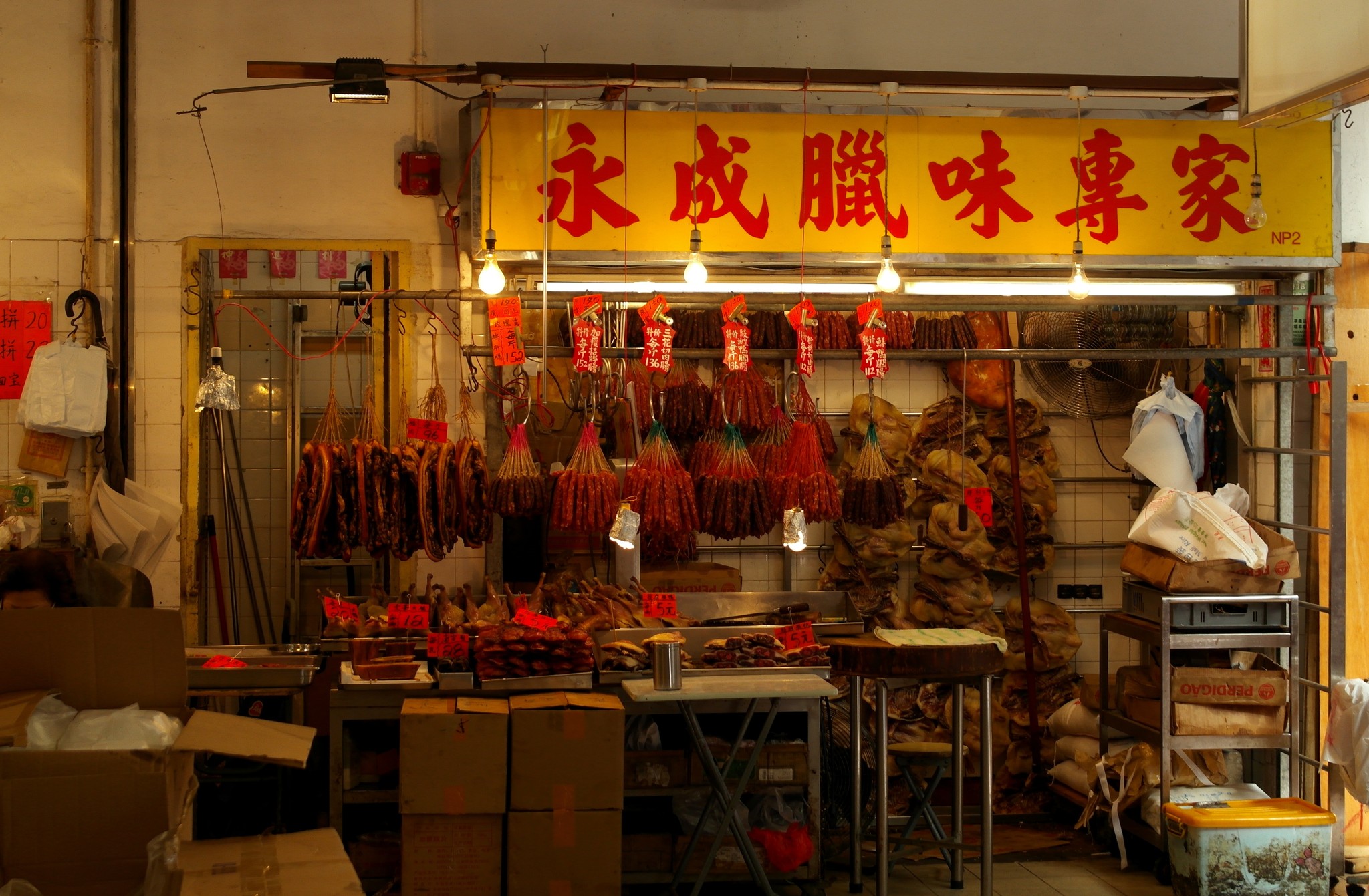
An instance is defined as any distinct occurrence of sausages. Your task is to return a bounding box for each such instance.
[612,307,978,350]
[488,362,907,562]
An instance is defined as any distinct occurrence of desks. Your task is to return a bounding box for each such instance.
[828,628,1005,896]
[327,687,822,894]
[622,673,841,896]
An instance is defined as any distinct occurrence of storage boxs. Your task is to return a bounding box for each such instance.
[1162,797,1337,895]
[642,559,742,598]
[1,606,365,896]
[627,741,807,876]
[397,691,623,896]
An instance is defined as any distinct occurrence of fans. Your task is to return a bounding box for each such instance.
[1024,310,1180,420]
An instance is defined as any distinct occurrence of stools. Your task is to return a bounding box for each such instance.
[886,742,959,881]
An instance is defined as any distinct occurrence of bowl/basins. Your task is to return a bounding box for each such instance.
[355,665,420,679]
[265,643,320,655]
[371,657,414,661]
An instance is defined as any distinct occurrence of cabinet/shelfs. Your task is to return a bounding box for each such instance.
[1095,595,1303,888]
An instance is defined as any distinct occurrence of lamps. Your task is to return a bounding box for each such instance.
[328,56,390,103]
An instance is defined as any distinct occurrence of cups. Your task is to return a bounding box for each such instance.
[652,642,683,691]
[386,643,415,657]
[344,640,383,673]
